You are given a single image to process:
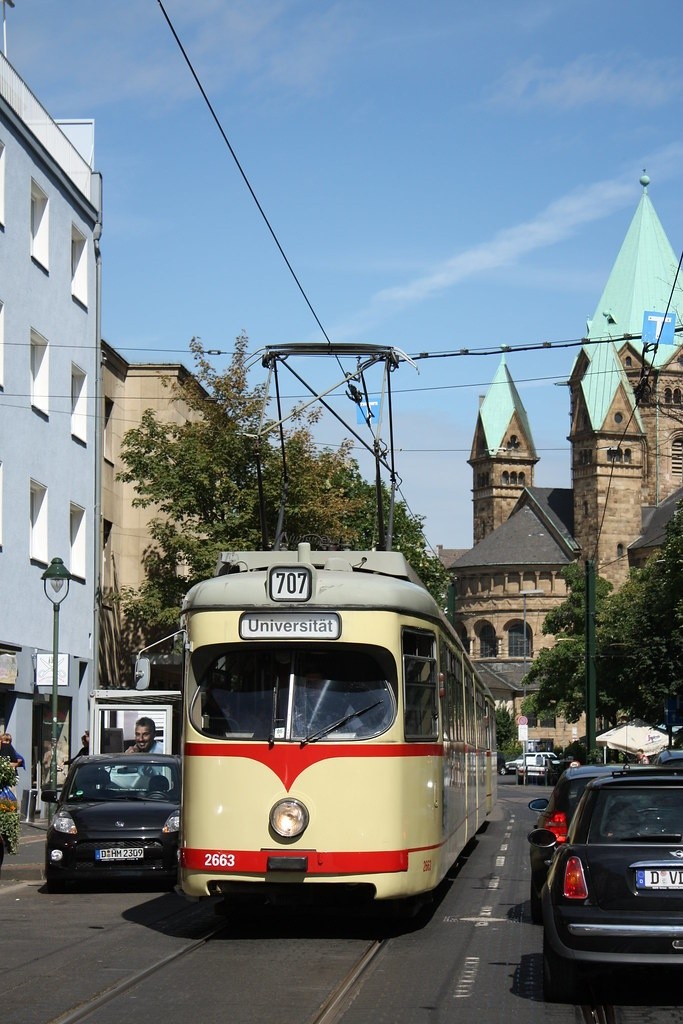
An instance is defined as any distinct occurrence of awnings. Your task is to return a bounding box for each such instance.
[580,718,676,763]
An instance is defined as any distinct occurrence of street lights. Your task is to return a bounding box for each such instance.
[41,557,73,826]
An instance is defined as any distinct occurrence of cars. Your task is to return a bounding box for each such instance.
[525,772,683,1000]
[657,749,682,766]
[525,762,683,922]
[497,750,561,785]
[40,752,181,891]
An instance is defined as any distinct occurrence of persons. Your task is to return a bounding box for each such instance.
[0,733,17,768]
[636,751,649,764]
[122,717,163,754]
[63,734,89,765]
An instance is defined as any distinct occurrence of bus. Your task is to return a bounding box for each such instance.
[133,543,495,925]
